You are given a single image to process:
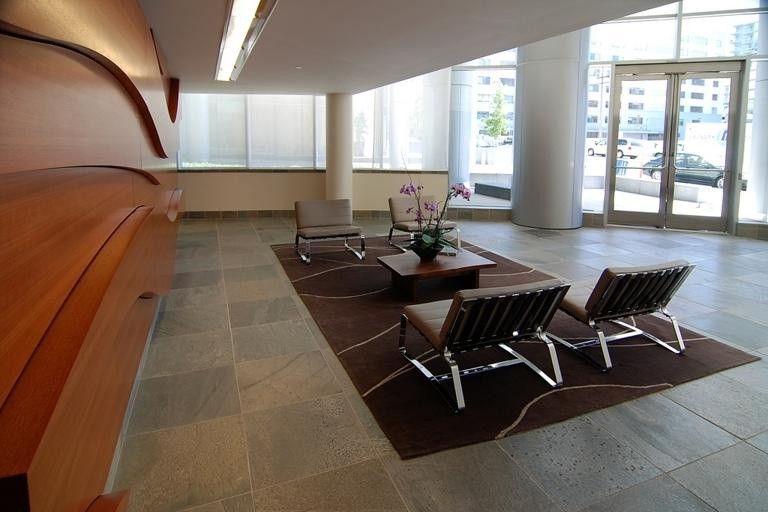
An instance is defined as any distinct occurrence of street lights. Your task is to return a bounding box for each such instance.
[599,74,609,142]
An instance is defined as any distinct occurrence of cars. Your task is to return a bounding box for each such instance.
[587,136,751,193]
[481,136,511,148]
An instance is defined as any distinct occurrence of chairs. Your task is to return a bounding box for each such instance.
[397,276,571,412]
[293,198,368,265]
[555,260,697,373]
[384,193,463,252]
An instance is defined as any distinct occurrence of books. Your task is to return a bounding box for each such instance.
[438,247,457,256]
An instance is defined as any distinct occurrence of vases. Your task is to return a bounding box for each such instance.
[404,236,441,262]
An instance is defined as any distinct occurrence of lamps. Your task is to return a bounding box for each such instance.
[213,0,277,84]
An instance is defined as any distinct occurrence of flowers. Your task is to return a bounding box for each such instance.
[391,178,477,249]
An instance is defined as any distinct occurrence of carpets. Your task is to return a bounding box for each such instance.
[270,231,763,460]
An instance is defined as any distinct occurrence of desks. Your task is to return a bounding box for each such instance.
[375,250,499,303]
